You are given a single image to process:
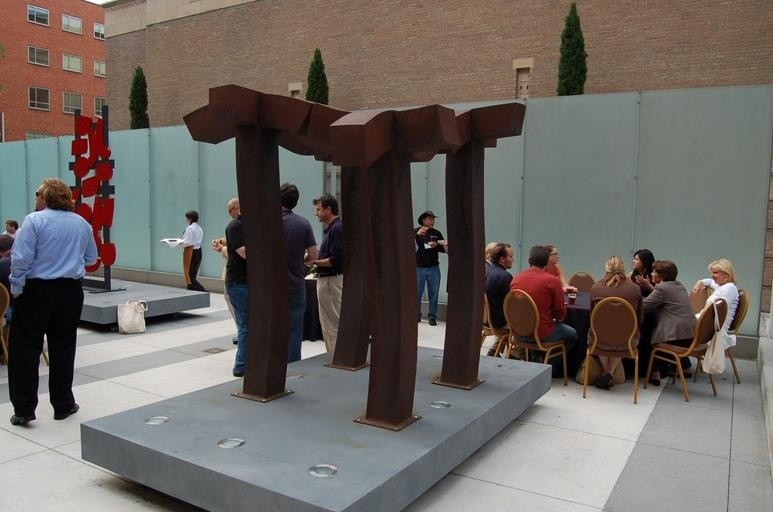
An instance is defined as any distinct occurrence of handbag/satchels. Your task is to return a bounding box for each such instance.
[703,300,737,375]
[114,298,148,334]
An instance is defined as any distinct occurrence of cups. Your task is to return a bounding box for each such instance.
[567,289,577,306]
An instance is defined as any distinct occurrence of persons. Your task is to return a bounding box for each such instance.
[547,247,578,294]
[10,178,98,423]
[487,241,501,267]
[414,210,448,326]
[2,220,21,238]
[486,245,514,359]
[173,210,205,291]
[308,194,343,355]
[281,184,319,362]
[225,216,246,378]
[642,261,695,386]
[628,248,656,296]
[509,246,577,381]
[1,234,11,365]
[694,260,739,375]
[587,256,642,390]
[211,198,242,342]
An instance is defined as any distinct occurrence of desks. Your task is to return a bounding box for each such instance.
[561,290,592,311]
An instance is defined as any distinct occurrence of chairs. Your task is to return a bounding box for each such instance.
[503,289,568,386]
[568,271,593,290]
[582,297,639,404]
[643,297,728,402]
[480,292,527,360]
[694,288,750,384]
[688,281,712,373]
[0,283,10,366]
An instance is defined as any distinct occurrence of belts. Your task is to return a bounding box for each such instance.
[313,272,342,279]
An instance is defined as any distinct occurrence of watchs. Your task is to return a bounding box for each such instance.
[312,259,318,266]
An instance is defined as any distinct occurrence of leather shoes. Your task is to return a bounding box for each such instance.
[428,318,438,326]
[649,377,660,386]
[677,372,693,378]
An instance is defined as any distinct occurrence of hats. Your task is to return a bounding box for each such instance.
[417,210,440,225]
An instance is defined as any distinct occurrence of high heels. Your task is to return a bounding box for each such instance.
[593,373,613,389]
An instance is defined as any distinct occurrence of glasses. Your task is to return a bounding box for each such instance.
[228,207,237,213]
[35,191,44,196]
[550,252,560,256]
